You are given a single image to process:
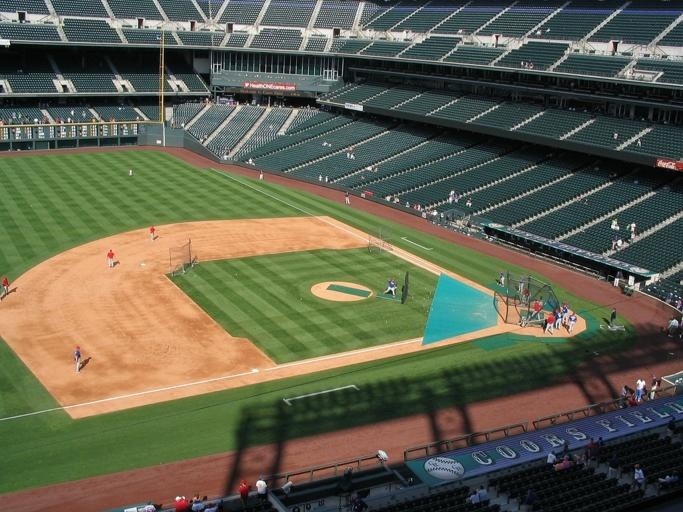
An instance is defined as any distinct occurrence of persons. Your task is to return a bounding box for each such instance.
[181,95,326,111]
[150,225,155,241]
[0,101,140,136]
[318,139,378,183]
[174,478,295,512]
[610,218,637,287]
[1,275,9,295]
[621,375,660,409]
[245,157,256,167]
[545,435,679,487]
[524,489,540,506]
[129,169,133,176]
[497,272,577,335]
[637,139,642,147]
[385,190,498,244]
[662,291,683,341]
[73,345,81,374]
[467,490,479,504]
[610,308,617,327]
[345,191,350,205]
[478,485,488,501]
[105,249,117,268]
[383,278,397,298]
[521,59,534,69]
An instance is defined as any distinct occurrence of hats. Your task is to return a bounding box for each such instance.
[175,496,181,501]
[634,464,641,468]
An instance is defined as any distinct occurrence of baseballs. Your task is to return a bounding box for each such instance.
[424,458,464,481]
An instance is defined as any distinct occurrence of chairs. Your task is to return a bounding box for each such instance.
[361,421,681,511]
